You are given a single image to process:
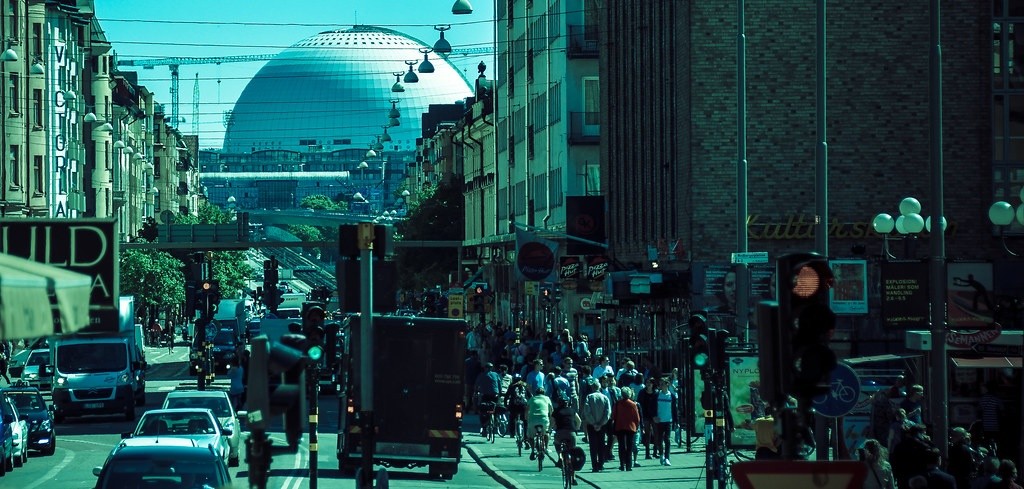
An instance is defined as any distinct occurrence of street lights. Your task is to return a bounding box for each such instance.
[874,197,946,393]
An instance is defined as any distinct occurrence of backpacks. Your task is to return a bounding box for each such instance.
[514,386,528,406]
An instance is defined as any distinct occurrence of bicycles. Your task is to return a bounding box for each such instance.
[146,329,173,355]
[474,392,575,489]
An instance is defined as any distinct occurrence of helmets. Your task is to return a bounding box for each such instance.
[558,395,569,401]
[603,369,614,377]
[513,374,521,379]
[484,362,494,368]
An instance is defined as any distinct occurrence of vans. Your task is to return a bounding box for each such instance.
[7,348,52,391]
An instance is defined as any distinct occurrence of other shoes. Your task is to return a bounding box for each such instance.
[626,468,632,471]
[660,454,665,465]
[653,454,660,459]
[664,459,671,466]
[645,455,652,459]
[619,467,624,471]
[633,463,641,467]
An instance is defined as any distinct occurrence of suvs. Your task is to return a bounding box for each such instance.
[0,387,56,455]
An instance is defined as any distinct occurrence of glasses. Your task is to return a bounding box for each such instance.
[538,364,542,365]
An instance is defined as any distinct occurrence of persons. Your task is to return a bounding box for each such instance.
[953,275,993,315]
[755,382,1024,489]
[714,269,776,332]
[150,319,176,352]
[227,350,252,412]
[189,419,207,434]
[0,340,26,385]
[61,349,90,372]
[466,320,678,486]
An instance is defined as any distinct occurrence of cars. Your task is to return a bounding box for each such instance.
[0,390,28,477]
[92,388,247,489]
[245,301,261,342]
[247,253,295,295]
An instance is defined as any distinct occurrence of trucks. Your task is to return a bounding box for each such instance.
[186,299,246,375]
[274,292,307,318]
[134,324,148,405]
[50,295,143,424]
[335,313,467,479]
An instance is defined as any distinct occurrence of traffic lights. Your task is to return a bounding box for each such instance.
[689,309,710,368]
[775,249,837,398]
[246,301,326,433]
[202,280,213,317]
[473,284,484,313]
